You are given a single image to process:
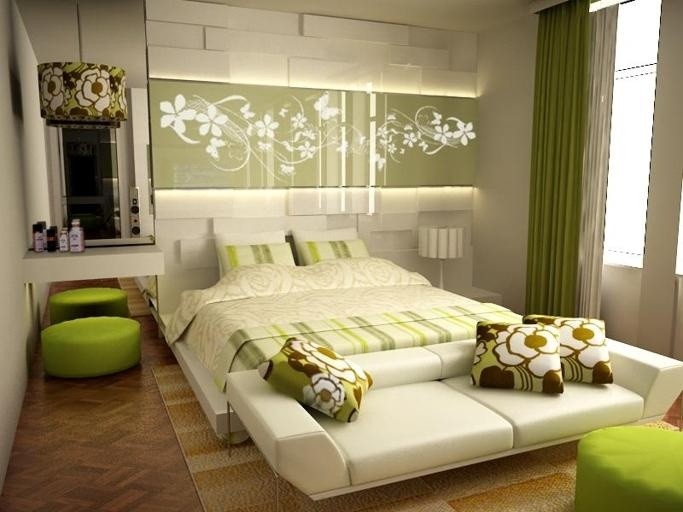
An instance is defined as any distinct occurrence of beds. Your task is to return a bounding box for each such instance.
[171,268,527,400]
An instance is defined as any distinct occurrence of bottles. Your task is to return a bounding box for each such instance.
[31,215,86,253]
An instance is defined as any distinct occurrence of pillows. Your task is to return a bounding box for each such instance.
[255,333,375,426]
[467,310,617,396]
[212,226,371,283]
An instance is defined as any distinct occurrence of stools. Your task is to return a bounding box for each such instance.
[571,423,682,511]
[39,314,143,378]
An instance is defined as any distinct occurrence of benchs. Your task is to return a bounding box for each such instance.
[220,328,682,504]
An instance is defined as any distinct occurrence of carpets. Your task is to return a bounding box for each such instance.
[148,359,679,511]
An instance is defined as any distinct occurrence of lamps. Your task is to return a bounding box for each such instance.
[415,224,465,289]
